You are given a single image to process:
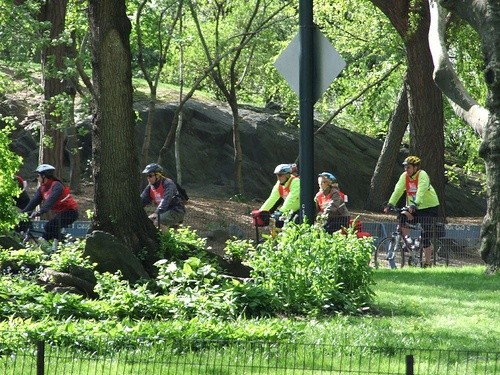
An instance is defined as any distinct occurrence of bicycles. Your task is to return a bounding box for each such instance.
[249,211,301,249]
[374,206,449,273]
[16,214,85,254]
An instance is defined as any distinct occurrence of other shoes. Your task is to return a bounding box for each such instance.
[401,240,415,251]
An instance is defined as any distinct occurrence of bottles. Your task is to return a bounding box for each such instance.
[414,236,421,247]
[38,237,49,246]
[405,235,415,249]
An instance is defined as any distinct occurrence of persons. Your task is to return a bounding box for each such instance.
[384,156,440,268]
[12,164,80,242]
[140,163,187,233]
[252,163,302,226]
[314,172,350,236]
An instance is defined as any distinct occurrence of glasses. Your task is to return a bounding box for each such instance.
[37,174,42,178]
[146,174,155,178]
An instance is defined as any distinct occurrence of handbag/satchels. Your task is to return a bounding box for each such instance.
[252,210,270,226]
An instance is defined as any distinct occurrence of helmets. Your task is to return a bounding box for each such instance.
[273,163,292,174]
[143,164,162,174]
[36,163,56,176]
[402,155,421,168]
[318,172,336,182]
